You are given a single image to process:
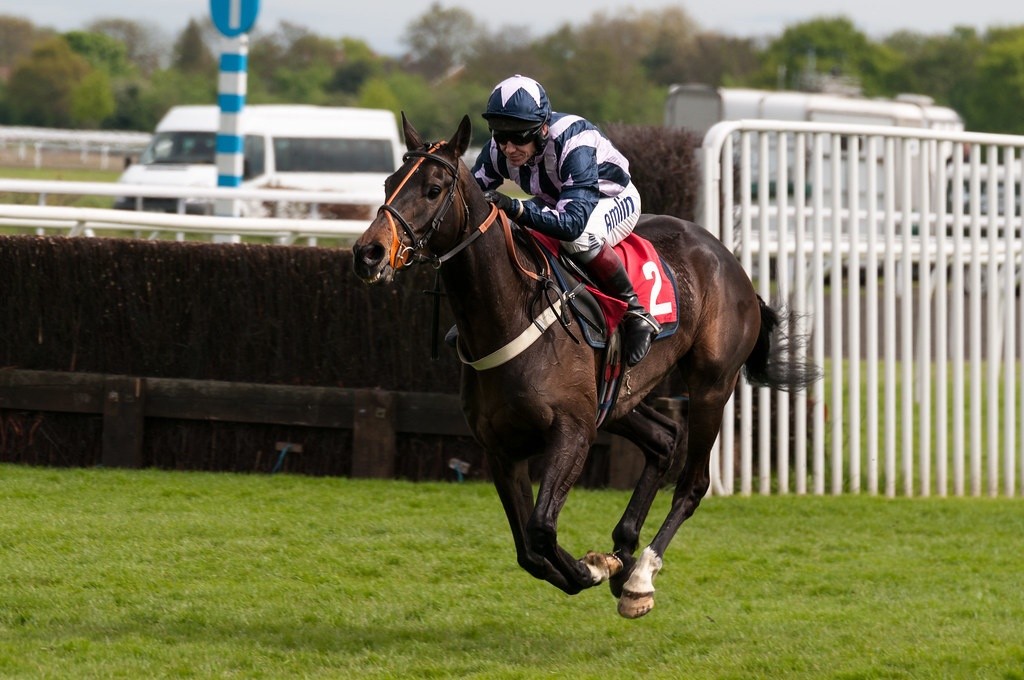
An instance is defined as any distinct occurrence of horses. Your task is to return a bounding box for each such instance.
[353,109,820,621]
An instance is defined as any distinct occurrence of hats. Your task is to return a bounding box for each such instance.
[481,74,552,133]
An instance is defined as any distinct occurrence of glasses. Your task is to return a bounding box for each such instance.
[493,128,540,145]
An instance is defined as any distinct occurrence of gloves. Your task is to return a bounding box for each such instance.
[482,189,512,218]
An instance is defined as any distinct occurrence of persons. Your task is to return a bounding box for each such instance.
[471,73,663,367]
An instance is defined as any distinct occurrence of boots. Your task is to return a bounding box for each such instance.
[583,238,663,368]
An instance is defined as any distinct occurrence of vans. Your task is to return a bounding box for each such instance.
[113,104,404,221]
